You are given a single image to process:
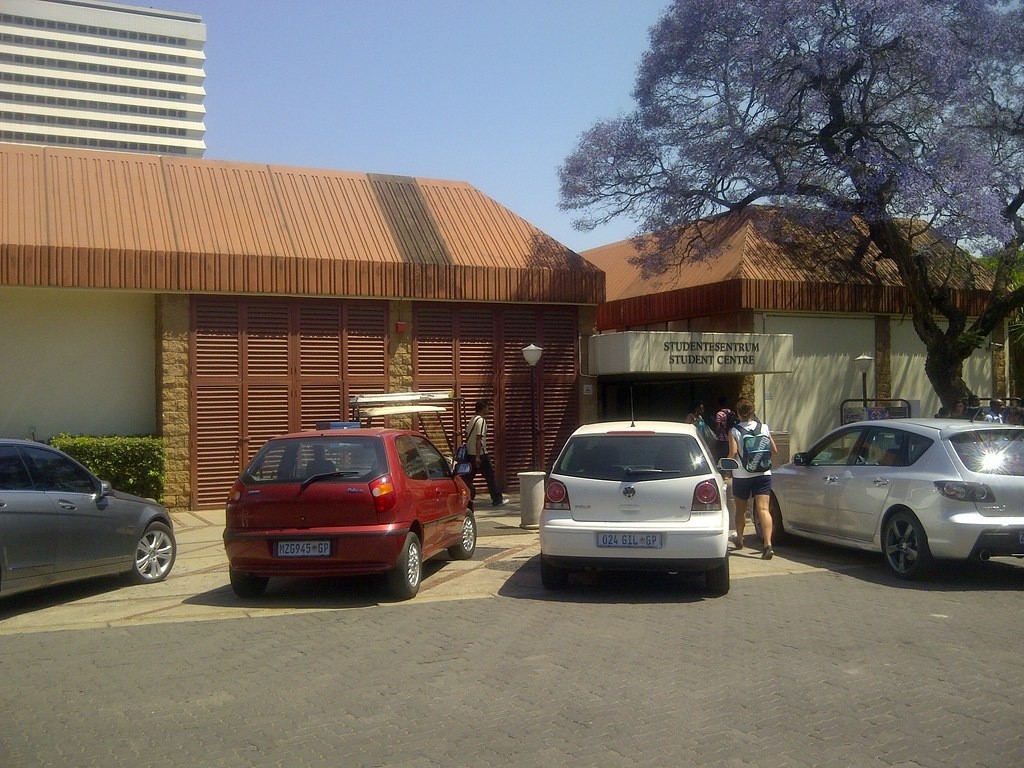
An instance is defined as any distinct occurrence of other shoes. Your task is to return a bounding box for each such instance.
[730,536,743,549]
[495,498,510,506]
[762,550,774,560]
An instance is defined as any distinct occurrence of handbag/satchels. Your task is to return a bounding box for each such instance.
[698,415,718,449]
[453,445,467,464]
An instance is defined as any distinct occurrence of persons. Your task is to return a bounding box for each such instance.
[685,397,777,560]
[934,395,1024,423]
[461,400,510,507]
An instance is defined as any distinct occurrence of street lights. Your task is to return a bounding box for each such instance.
[522,343,546,470]
[856,354,874,407]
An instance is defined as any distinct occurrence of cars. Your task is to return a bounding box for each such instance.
[750,418,1024,577]
[539,422,742,598]
[1,438,177,601]
[221,420,476,600]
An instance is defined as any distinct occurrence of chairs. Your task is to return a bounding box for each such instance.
[657,444,689,473]
[585,445,619,476]
[305,460,335,478]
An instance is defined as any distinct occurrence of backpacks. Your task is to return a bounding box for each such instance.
[733,421,772,473]
